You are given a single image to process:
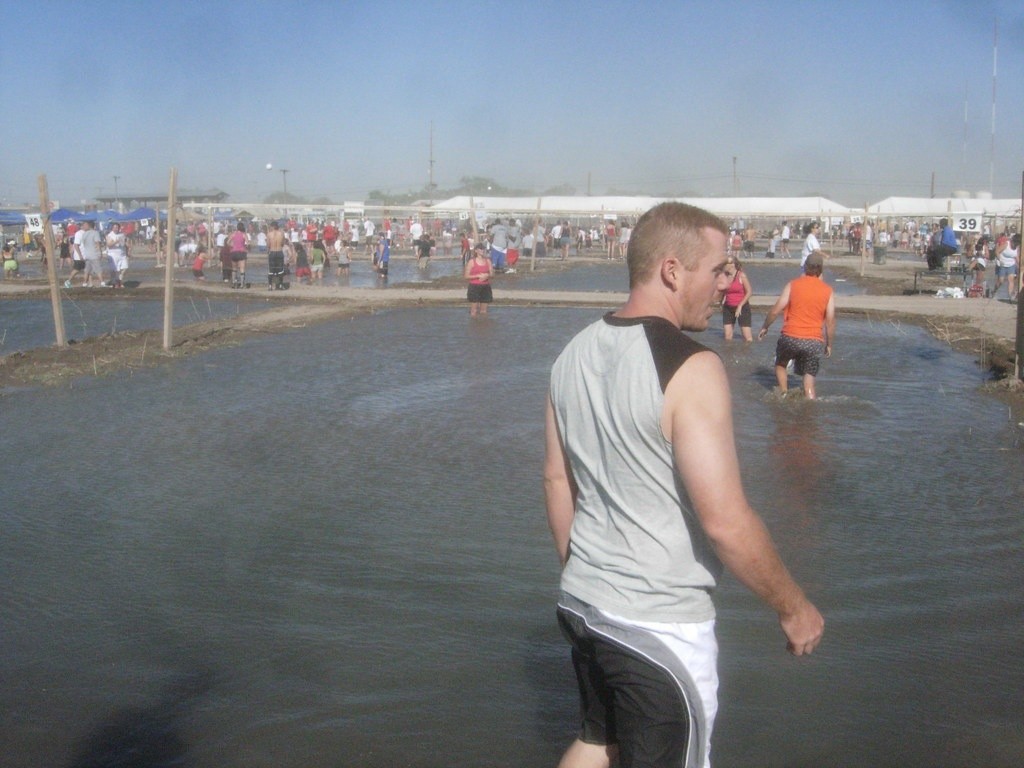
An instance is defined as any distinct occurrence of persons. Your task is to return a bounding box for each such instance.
[840,217,1021,302]
[757,252,836,400]
[0,213,633,291]
[543,199,826,768]
[727,217,840,280]
[465,244,495,314]
[723,256,753,343]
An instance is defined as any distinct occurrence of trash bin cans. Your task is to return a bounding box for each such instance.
[873,246,887,265]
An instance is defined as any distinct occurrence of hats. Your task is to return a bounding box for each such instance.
[474,243,486,249]
[806,254,824,266]
[270,222,277,226]
[379,232,384,236]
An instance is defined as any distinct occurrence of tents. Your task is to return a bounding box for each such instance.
[0,207,169,224]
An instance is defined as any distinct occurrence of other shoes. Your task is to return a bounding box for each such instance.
[268,285,272,290]
[280,284,286,289]
[780,255,784,259]
[788,255,792,258]
[505,268,516,274]
[608,258,617,260]
[620,256,626,260]
[65,280,125,288]
